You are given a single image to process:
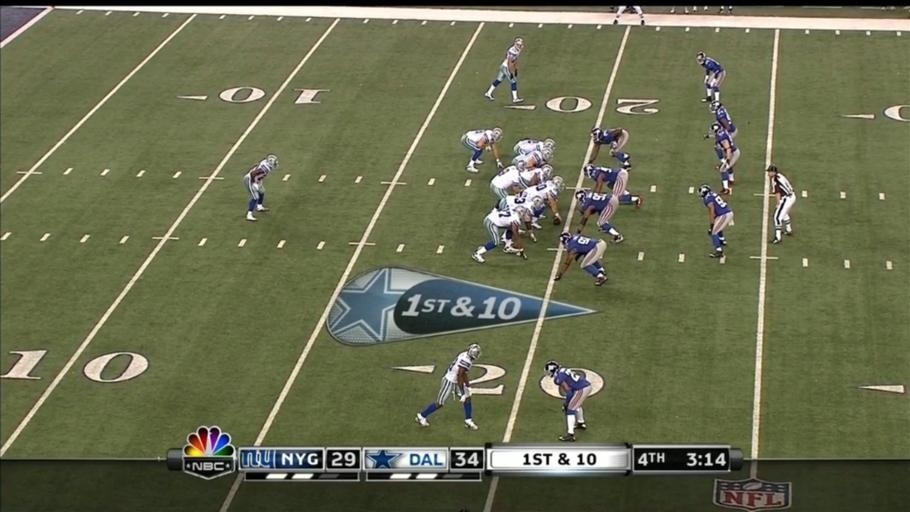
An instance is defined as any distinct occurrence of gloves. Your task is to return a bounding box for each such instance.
[710,79,716,88]
[459,394,466,404]
[496,161,504,169]
[511,73,513,79]
[555,275,561,281]
[253,182,261,190]
[704,75,709,85]
[466,387,473,398]
[515,70,517,77]
[562,402,568,412]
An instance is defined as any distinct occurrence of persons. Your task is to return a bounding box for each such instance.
[696,183,735,258]
[612,5,646,26]
[242,154,280,221]
[543,359,594,443]
[483,38,525,104]
[459,125,646,287]
[413,343,482,430]
[696,50,726,103]
[764,165,797,244]
[708,100,738,140]
[710,121,741,195]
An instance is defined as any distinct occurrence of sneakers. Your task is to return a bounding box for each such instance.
[770,237,782,244]
[641,21,644,25]
[782,229,793,235]
[485,92,495,101]
[257,207,266,211]
[513,98,525,103]
[246,214,257,221]
[475,159,483,165]
[575,423,586,430]
[414,412,430,426]
[594,153,642,285]
[614,20,618,25]
[701,98,737,258]
[467,165,479,173]
[558,432,576,441]
[472,222,542,263]
[464,418,479,430]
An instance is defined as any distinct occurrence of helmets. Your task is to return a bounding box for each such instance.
[544,359,560,378]
[696,52,706,65]
[575,189,586,204]
[266,154,279,170]
[515,37,524,52]
[697,184,711,198]
[584,163,596,179]
[491,128,503,144]
[560,231,572,246]
[467,343,481,360]
[590,127,603,143]
[514,138,565,219]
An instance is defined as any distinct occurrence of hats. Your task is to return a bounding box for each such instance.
[765,165,777,172]
